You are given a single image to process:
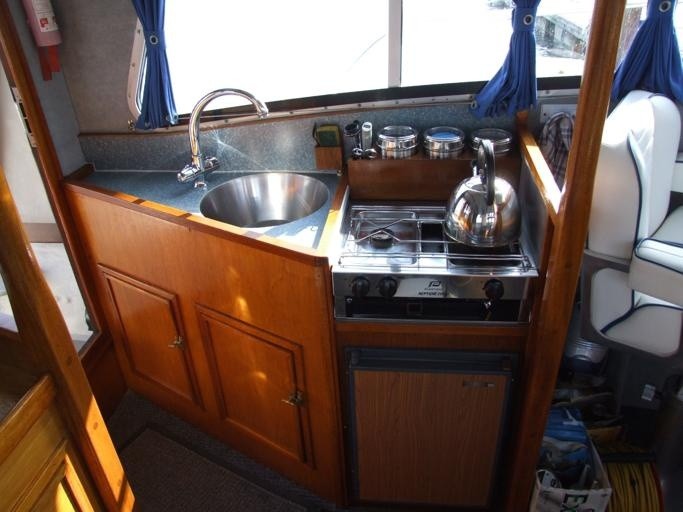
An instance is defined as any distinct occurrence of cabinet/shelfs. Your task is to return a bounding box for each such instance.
[96,264,203,410]
[194,303,316,468]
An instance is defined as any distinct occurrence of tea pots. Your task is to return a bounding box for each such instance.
[441,140,522,249]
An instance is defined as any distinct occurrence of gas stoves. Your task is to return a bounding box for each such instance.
[331,203,538,323]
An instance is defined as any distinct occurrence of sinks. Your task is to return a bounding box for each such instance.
[199,171,329,229]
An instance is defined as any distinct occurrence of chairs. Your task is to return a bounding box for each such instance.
[345,343,519,511]
[582,87,683,357]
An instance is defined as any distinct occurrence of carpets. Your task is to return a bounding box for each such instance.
[118,424,316,512]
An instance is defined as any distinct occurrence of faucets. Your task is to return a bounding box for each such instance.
[177,88,269,190]
